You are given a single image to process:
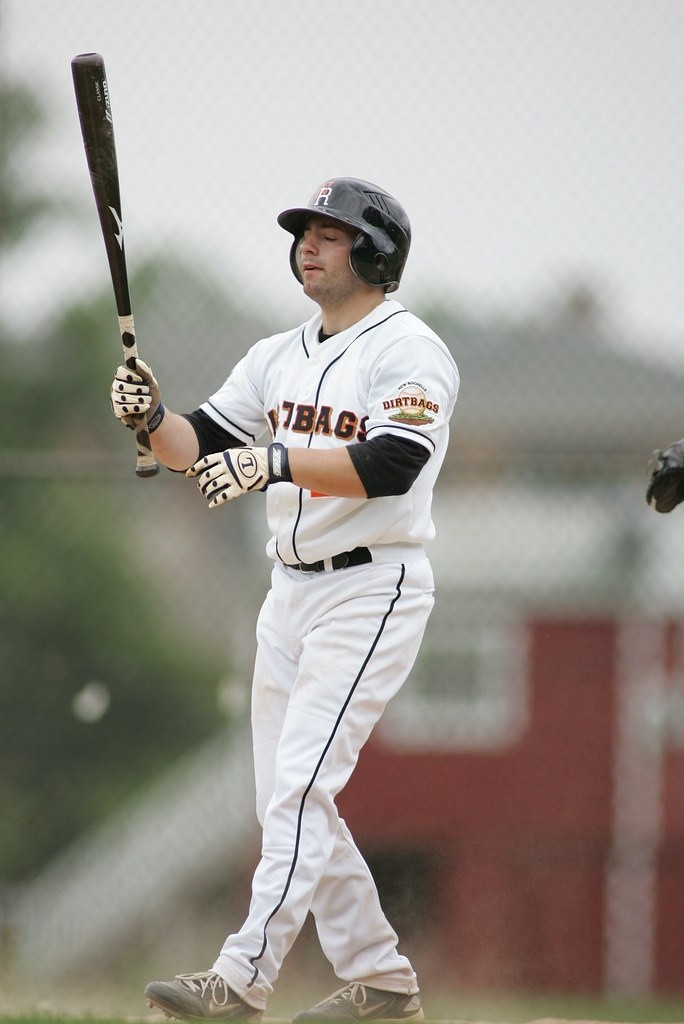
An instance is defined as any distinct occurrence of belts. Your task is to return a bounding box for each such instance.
[284,545,372,573]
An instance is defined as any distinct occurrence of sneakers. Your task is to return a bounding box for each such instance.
[144,969,265,1024]
[290,981,425,1024]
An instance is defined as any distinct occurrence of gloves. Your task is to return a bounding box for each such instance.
[186,442,293,508]
[109,359,164,434]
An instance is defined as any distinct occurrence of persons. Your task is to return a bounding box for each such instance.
[110,179,462,1022]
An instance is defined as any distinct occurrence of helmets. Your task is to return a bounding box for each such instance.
[277,177,412,292]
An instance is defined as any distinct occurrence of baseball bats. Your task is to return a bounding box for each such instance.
[70,51,163,479]
